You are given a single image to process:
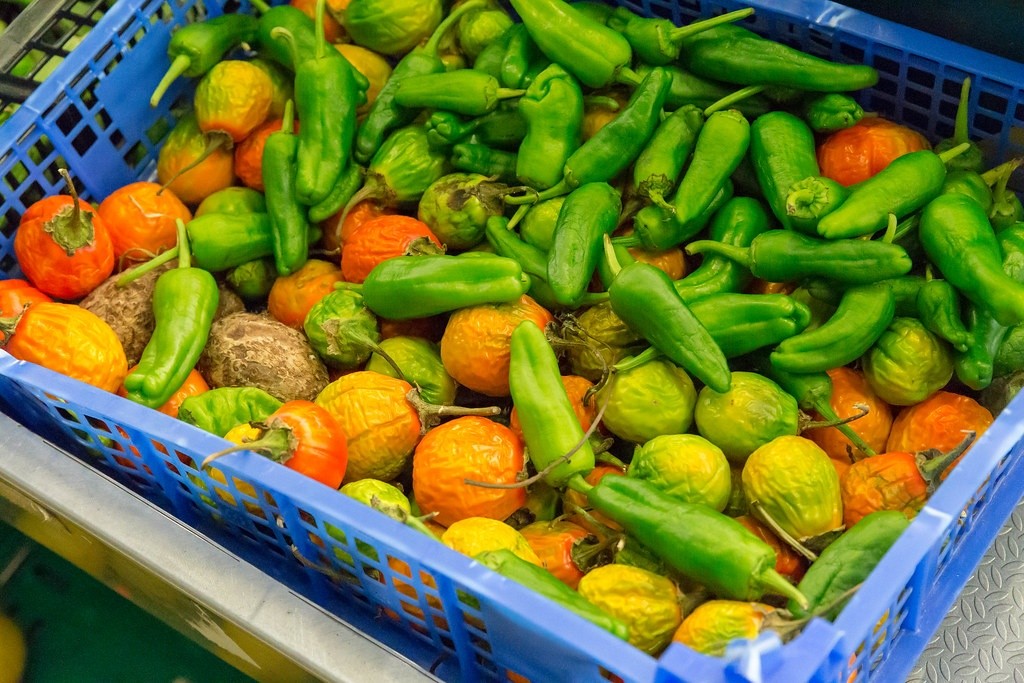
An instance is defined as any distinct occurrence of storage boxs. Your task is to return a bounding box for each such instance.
[0,0,1024,683]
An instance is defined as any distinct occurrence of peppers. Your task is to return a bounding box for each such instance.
[0,0,1024,658]
